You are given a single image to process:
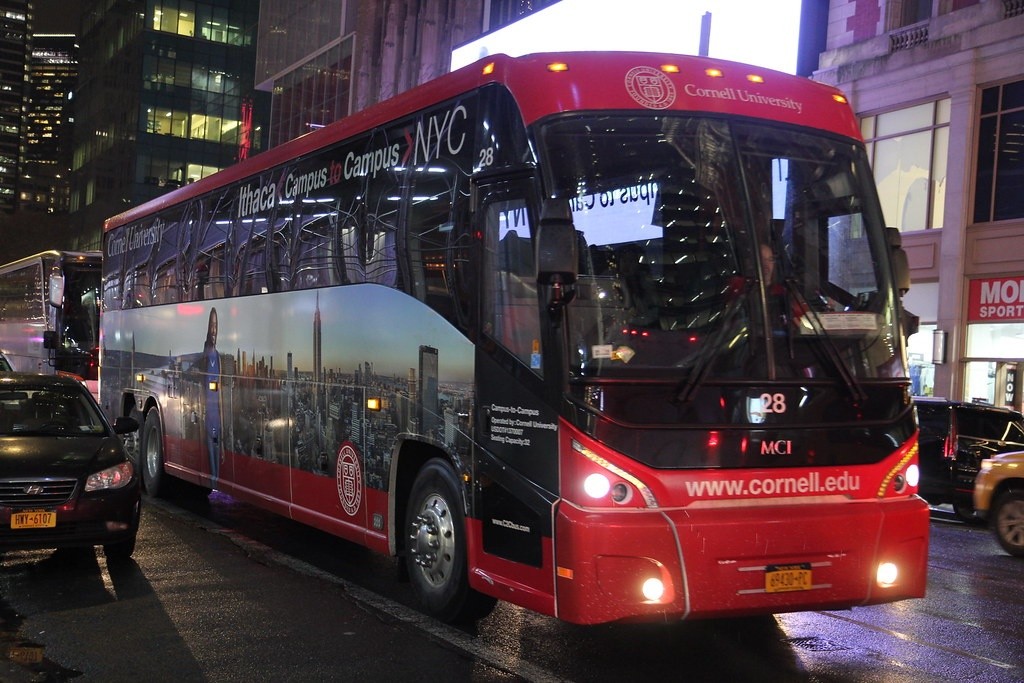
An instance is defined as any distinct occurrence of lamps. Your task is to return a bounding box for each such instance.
[932,328,946,365]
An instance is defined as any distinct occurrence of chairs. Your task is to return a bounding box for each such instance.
[17,398,51,430]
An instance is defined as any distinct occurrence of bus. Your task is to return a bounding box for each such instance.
[92,52,929,625]
[0,249,102,403]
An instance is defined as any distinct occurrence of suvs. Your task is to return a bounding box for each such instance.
[913,396,1023,521]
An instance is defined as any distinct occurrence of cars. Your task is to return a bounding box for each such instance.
[0,371,139,565]
[974,452,1024,561]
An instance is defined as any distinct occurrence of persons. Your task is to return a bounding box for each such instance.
[726,243,834,325]
[200,306,225,489]
